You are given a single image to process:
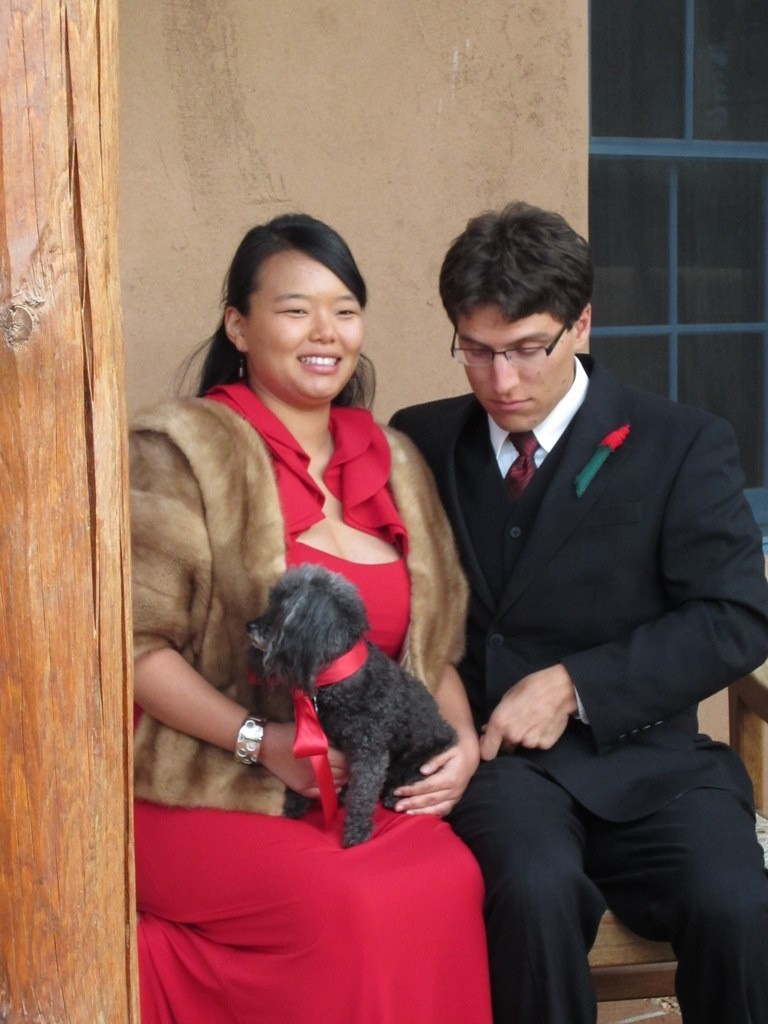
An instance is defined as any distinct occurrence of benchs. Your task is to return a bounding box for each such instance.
[580,659,768,1005]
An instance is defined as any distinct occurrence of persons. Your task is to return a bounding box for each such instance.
[129,209,496,1024]
[384,201,767,1024]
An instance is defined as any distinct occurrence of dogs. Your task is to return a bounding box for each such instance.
[242,561,458,849]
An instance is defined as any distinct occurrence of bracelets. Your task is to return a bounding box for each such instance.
[235,709,269,767]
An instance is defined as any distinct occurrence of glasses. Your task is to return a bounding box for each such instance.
[449,321,570,364]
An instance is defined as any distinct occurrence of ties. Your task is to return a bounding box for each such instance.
[505,432,540,507]
